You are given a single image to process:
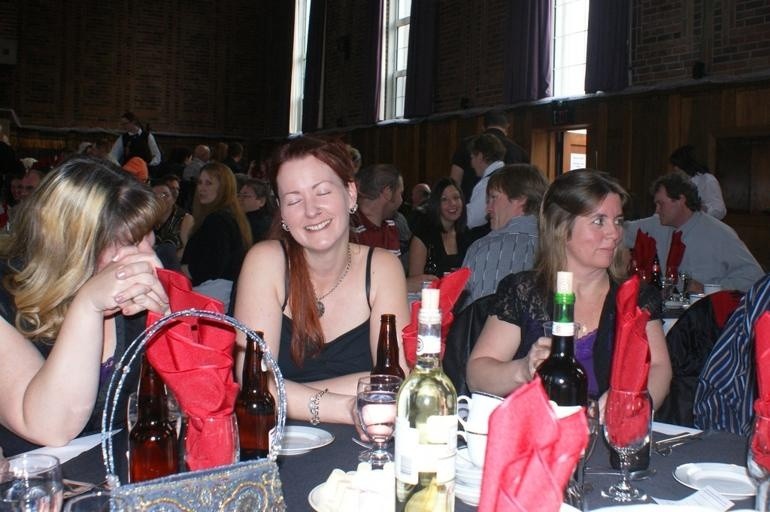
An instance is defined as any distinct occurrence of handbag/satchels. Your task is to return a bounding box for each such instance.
[102,309,291,512]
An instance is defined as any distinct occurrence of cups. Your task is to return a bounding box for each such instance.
[704,282,722,293]
[457,392,507,433]
[458,434,487,468]
[542,321,579,357]
[2,454,62,512]
[603,388,651,501]
[745,417,770,512]
[357,375,396,464]
[689,294,707,305]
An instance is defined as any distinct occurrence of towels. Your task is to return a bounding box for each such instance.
[0,201,10,228]
[631,227,658,281]
[146,265,238,469]
[753,311,770,471]
[607,275,651,446]
[478,374,590,512]
[402,268,470,373]
[666,231,687,285]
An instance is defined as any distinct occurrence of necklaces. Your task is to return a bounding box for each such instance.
[317,244,351,317]
[579,281,610,336]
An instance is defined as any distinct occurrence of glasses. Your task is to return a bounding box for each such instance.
[237,193,258,199]
[655,198,678,206]
[121,122,130,125]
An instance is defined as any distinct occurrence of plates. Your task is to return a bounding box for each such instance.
[672,463,759,500]
[666,301,683,310]
[308,469,396,512]
[276,426,336,454]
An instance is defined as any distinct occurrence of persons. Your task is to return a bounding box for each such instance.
[460,163,550,311]
[107,112,161,166]
[464,169,672,428]
[151,182,193,260]
[347,163,438,293]
[408,177,471,277]
[121,139,152,181]
[155,142,192,177]
[179,162,252,314]
[466,133,505,241]
[451,108,531,203]
[92,137,112,160]
[185,144,209,176]
[0,155,171,448]
[623,172,766,293]
[232,133,411,444]
[10,176,23,201]
[238,178,272,241]
[671,142,727,220]
[693,270,770,434]
[223,142,242,172]
[162,174,180,200]
[412,183,431,213]
[16,169,44,202]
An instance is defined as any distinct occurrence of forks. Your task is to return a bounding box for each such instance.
[655,437,702,456]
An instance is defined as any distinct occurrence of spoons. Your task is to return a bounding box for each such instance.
[584,468,657,479]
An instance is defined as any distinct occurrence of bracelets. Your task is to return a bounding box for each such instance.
[309,387,331,426]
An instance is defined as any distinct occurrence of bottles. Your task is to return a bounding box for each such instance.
[370,313,407,399]
[129,355,179,485]
[533,270,589,409]
[648,258,662,293]
[234,330,277,463]
[393,289,457,512]
